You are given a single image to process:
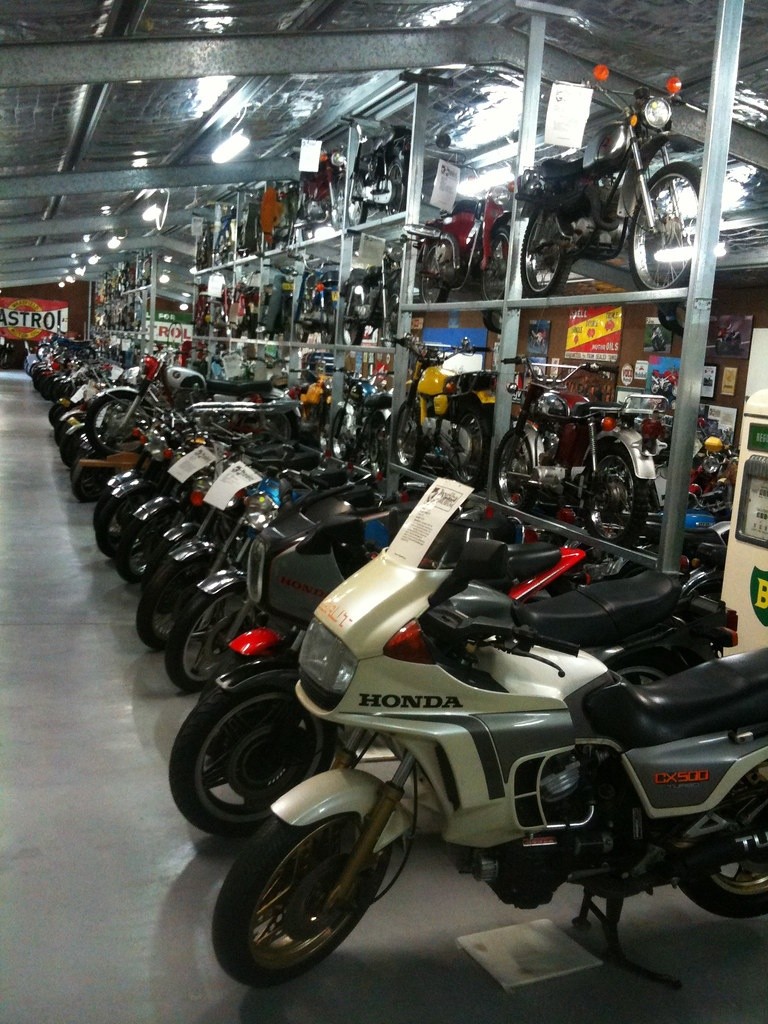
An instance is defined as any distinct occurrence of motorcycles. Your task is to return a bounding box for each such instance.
[514,64,702,300]
[403,189,511,303]
[24,330,736,837]
[208,535,768,992]
[92,115,409,350]
